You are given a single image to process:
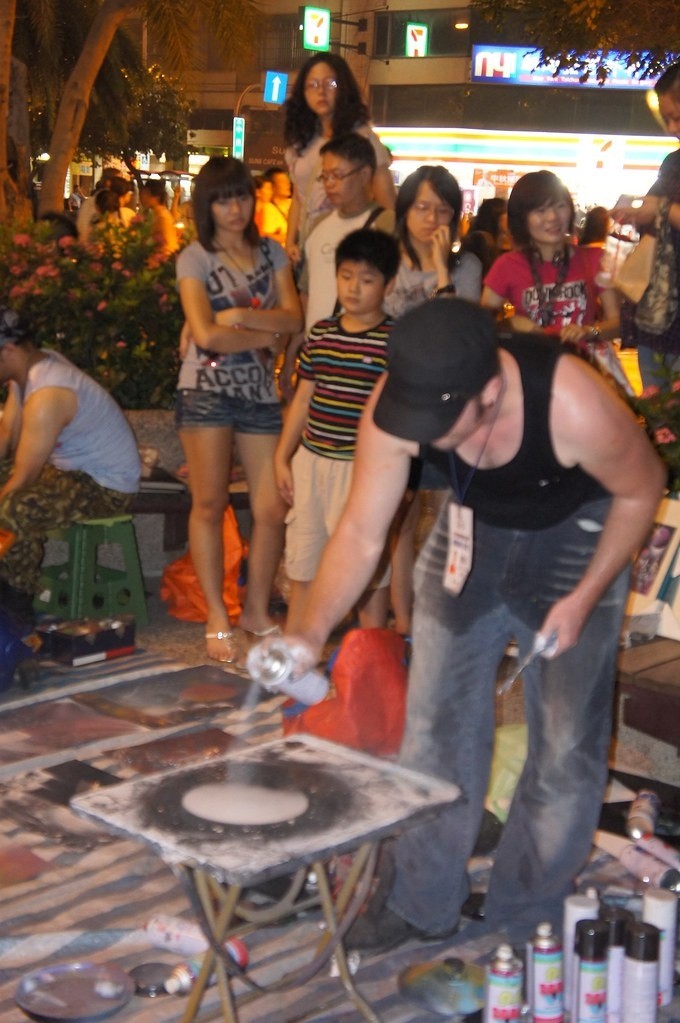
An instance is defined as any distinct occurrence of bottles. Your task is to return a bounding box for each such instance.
[485,877,677,1023]
[163,935,252,996]
[620,845,680,890]
[635,832,680,872]
[245,638,331,708]
[626,789,660,840]
[143,912,211,956]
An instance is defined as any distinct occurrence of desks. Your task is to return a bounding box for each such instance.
[70,733,466,1023]
[617,639,680,759]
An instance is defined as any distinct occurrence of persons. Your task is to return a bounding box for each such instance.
[176,155,303,665]
[484,170,621,360]
[272,227,414,651]
[68,167,198,344]
[0,306,141,661]
[260,295,669,1005]
[577,206,611,251]
[279,51,461,666]
[460,197,512,291]
[256,166,293,247]
[613,63,680,498]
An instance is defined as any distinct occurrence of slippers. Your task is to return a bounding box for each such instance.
[206,627,238,663]
[257,624,283,644]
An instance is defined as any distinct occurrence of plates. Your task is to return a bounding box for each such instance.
[15,960,135,1023]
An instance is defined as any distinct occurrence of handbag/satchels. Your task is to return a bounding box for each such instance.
[302,628,410,758]
[636,198,677,334]
[614,233,657,303]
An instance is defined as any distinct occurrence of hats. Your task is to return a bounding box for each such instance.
[372,296,499,444]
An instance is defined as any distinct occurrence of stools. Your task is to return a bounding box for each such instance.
[33,514,148,624]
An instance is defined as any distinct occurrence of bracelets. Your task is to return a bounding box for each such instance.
[589,324,601,338]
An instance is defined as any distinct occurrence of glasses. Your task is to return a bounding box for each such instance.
[313,164,366,185]
[301,77,337,93]
[412,200,455,222]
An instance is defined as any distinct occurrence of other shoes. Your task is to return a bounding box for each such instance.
[398,935,526,1016]
[340,908,459,954]
